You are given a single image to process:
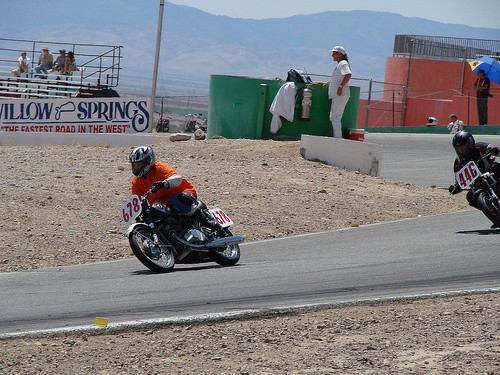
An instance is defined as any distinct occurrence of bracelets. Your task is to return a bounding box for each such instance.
[339,86,342,87]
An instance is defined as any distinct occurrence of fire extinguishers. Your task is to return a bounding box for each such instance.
[295,84,314,120]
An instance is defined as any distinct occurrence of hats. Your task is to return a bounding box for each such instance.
[59,49,65,53]
[330,46,345,55]
[42,46,49,51]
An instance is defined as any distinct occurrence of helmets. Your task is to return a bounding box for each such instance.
[426,117,438,125]
[453,132,476,161]
[129,146,156,178]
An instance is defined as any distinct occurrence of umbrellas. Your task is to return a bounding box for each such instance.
[469,57,500,87]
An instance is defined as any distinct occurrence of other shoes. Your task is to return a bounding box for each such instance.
[204,220,220,229]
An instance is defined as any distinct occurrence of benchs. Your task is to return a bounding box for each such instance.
[0,66,113,101]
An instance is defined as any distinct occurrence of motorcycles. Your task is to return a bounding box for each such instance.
[185,113,208,133]
[451,151,500,226]
[121,182,247,271]
[157,112,171,132]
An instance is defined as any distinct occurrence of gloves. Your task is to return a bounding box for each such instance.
[151,181,164,190]
[489,146,499,155]
[449,185,461,194]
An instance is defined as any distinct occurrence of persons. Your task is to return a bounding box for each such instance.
[129,146,228,236]
[448,130,500,206]
[475,69,490,125]
[322,46,352,138]
[449,114,463,134]
[35,47,54,79]
[62,51,76,74]
[54,49,66,71]
[17,52,30,77]
